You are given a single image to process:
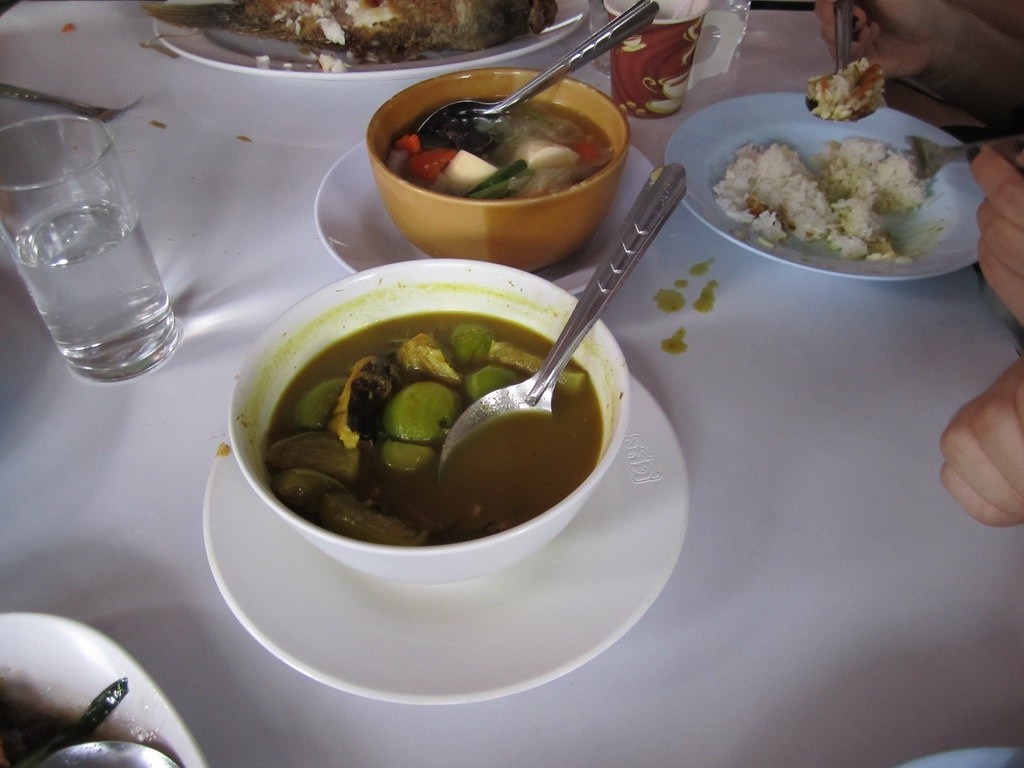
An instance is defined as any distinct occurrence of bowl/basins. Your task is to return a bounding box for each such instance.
[228,258,630,585]
[366,67,632,273]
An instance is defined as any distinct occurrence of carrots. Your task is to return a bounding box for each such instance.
[395,135,458,181]
[575,138,600,162]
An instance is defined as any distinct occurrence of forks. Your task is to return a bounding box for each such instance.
[903,135,1024,181]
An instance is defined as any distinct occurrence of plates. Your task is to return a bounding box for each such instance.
[663,93,986,281]
[1,611,210,767]
[314,140,655,295]
[897,746,1024,768]
[153,0,590,80]
[202,372,688,704]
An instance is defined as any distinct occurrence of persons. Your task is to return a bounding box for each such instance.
[940,352,1024,528]
[815,0,1024,327]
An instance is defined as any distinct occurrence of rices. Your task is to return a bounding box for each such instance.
[711,58,931,265]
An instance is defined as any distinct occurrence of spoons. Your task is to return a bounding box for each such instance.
[40,742,180,768]
[417,0,659,160]
[805,0,884,122]
[438,163,687,489]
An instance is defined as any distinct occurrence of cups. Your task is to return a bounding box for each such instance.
[603,1,710,121]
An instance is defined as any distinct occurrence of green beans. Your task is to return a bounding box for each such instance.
[462,158,534,200]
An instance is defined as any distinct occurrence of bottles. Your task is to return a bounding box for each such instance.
[0,115,180,382]
